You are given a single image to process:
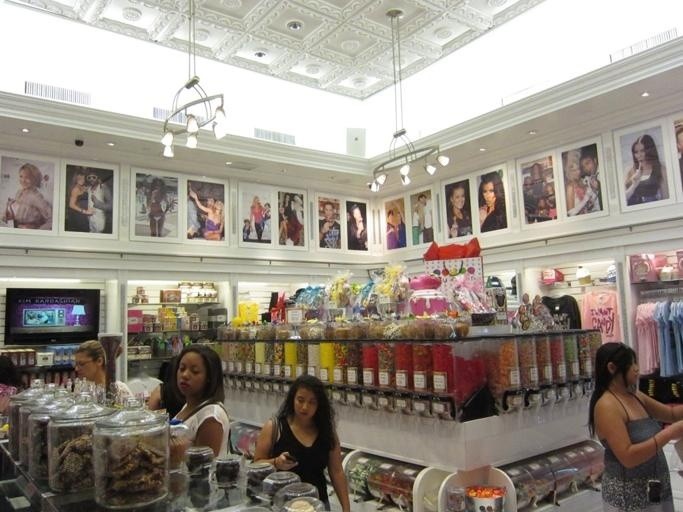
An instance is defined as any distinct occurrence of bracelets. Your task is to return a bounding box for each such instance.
[320,231,324,235]
[273,457,279,469]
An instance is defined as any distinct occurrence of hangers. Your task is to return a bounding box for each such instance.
[638,281,683,302]
[590,278,609,296]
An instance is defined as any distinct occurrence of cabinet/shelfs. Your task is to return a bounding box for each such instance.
[19,365,78,392]
[128,303,222,362]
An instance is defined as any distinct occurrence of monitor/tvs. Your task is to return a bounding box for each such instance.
[4,287,100,345]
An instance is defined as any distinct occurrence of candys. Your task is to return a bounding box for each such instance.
[216,295,603,419]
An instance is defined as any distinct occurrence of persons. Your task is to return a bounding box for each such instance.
[279,193,304,246]
[387,124,669,250]
[587,340,683,512]
[5,163,52,231]
[189,190,224,241]
[75,339,134,399]
[169,345,230,463]
[254,374,351,512]
[69,169,112,234]
[0,355,25,415]
[146,178,170,238]
[319,200,341,248]
[242,196,271,242]
[348,204,367,248]
[149,355,187,419]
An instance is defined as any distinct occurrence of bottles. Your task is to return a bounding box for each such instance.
[8,379,169,510]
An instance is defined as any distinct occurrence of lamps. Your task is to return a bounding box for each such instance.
[368,8,450,193]
[161,0,227,157]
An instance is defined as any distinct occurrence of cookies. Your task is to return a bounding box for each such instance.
[8,398,167,506]
